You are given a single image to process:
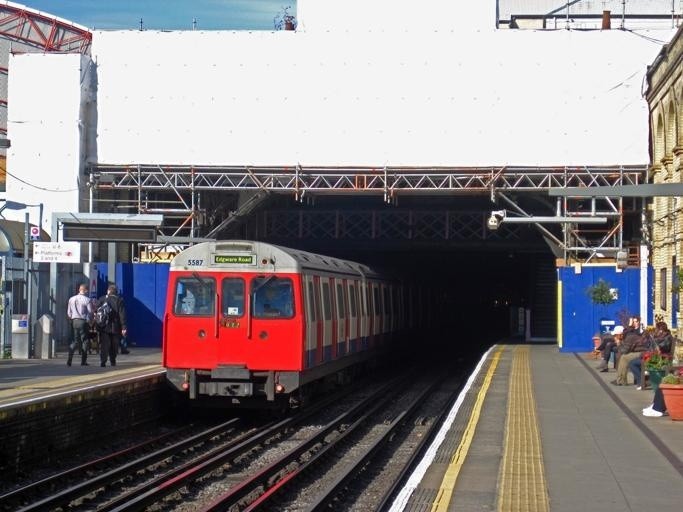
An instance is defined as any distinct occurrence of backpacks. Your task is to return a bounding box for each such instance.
[95,303,112,329]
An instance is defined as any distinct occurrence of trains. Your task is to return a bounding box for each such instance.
[161,239,442,411]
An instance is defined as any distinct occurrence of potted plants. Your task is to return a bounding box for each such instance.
[586,276,616,356]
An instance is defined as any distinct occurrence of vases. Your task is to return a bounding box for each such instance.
[658,383,683,421]
[650,366,665,392]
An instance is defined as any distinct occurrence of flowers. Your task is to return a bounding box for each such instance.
[644,351,671,371]
[661,365,683,383]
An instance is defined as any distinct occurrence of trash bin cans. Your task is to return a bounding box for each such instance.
[34,314,53,360]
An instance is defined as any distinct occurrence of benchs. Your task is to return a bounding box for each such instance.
[77,324,101,354]
[609,323,644,368]
[633,336,676,390]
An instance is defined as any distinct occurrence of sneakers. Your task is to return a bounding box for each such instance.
[637,385,642,389]
[642,403,663,417]
[611,380,622,386]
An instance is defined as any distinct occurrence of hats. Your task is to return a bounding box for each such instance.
[611,326,625,335]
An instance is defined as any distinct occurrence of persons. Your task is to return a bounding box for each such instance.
[66,283,95,367]
[584,313,675,418]
[93,283,127,367]
[119,328,129,354]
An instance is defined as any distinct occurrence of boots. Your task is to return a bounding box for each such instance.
[595,359,607,370]
[600,368,609,372]
